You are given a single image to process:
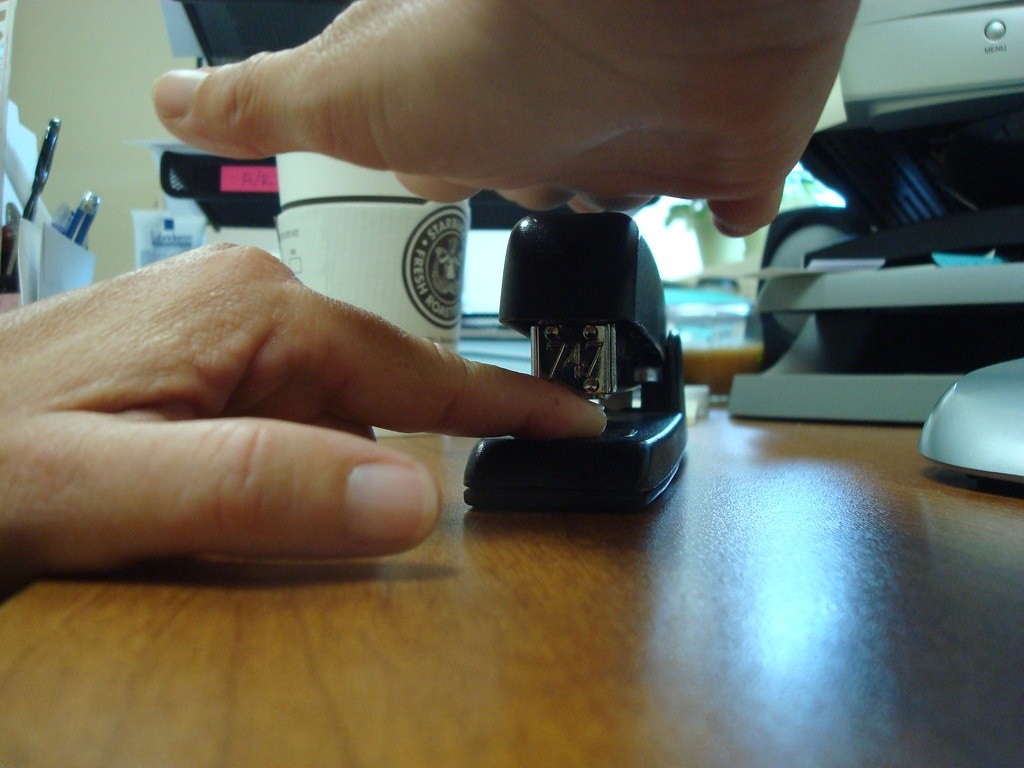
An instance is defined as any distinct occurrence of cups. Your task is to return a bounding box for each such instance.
[274,145,470,438]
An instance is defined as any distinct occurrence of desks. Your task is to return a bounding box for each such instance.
[1,408,1022,768]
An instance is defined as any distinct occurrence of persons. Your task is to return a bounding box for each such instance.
[1,0,867,558]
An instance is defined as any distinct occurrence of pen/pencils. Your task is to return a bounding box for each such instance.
[1,117,102,292]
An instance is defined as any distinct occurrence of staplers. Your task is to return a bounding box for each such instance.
[459,209,689,519]
